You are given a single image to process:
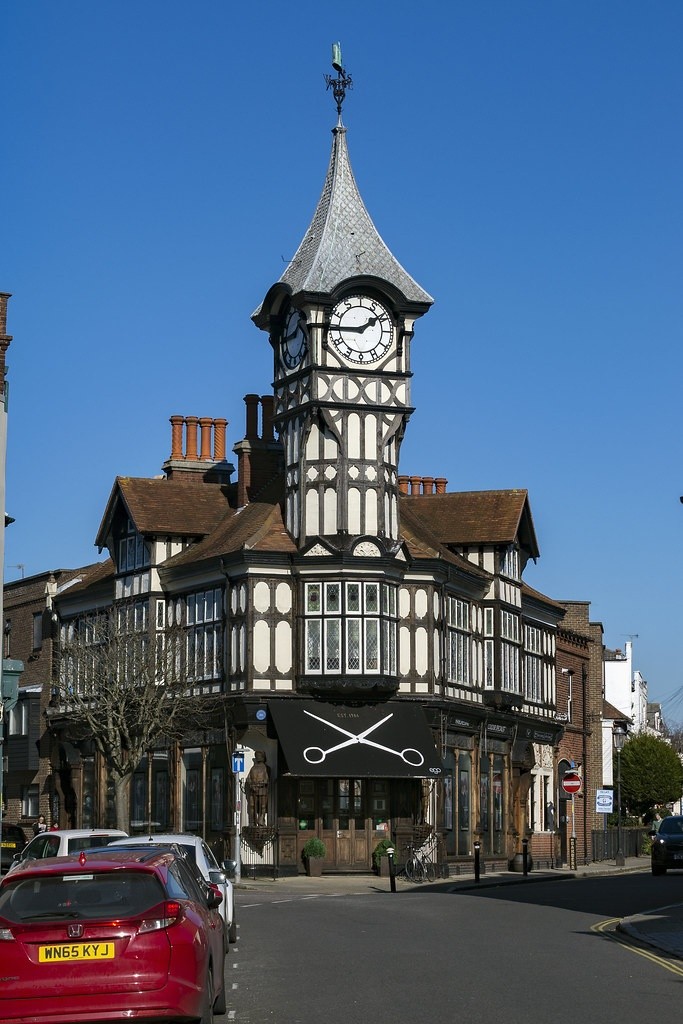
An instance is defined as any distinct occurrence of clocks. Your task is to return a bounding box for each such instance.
[329,294,394,364]
[280,305,311,370]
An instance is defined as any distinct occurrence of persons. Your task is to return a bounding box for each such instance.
[50,823,59,845]
[651,813,663,840]
[32,814,48,845]
[247,750,271,827]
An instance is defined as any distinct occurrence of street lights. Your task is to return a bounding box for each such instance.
[612,726,627,866]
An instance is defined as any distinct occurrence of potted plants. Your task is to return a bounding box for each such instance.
[372,839,399,878]
[302,837,327,878]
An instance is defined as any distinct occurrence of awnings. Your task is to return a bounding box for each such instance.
[267,697,450,779]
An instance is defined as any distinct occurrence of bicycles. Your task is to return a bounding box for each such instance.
[405,844,436,883]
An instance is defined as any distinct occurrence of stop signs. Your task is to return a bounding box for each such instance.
[562,774,581,793]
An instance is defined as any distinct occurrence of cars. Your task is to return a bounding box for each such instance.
[108,834,238,941]
[11,828,129,867]
[1,844,228,1022]
[649,815,683,877]
